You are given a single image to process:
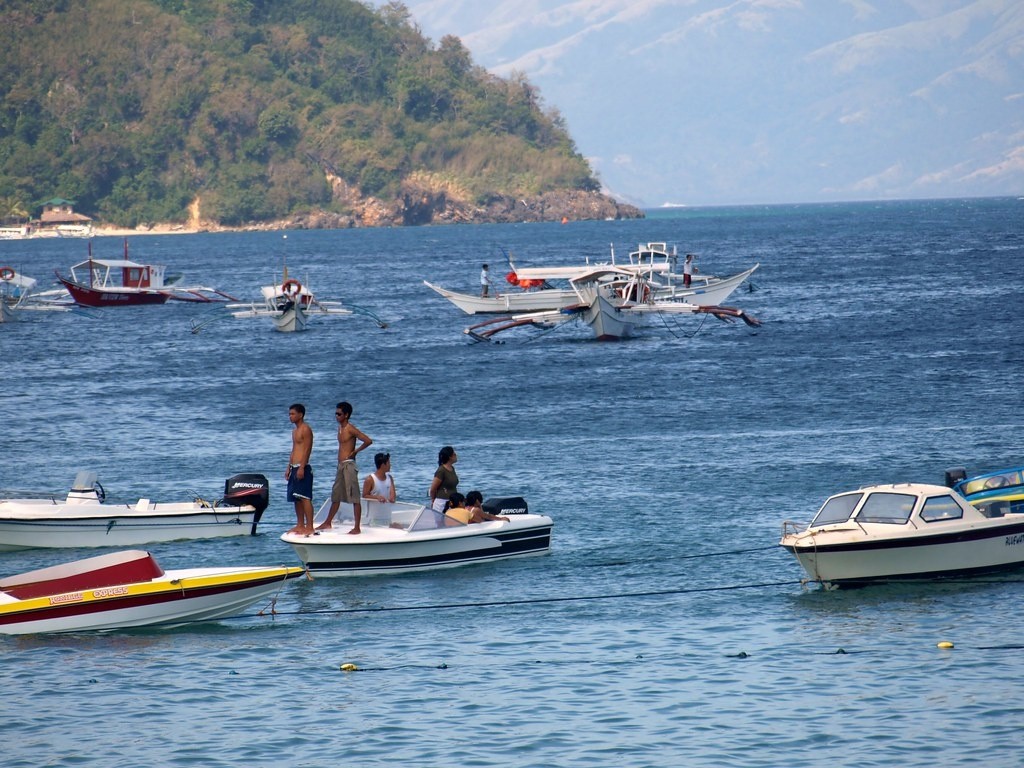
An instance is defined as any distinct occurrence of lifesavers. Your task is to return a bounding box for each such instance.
[0,266,15,281]
[281,280,302,297]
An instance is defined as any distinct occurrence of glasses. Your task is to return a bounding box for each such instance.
[383,453,387,462]
[335,412,347,417]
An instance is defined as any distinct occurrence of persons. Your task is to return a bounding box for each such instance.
[481,264,492,297]
[283,283,297,314]
[442,490,510,527]
[612,277,651,304]
[362,452,396,503]
[682,254,693,288]
[315,402,372,534]
[429,446,459,528]
[284,404,315,535]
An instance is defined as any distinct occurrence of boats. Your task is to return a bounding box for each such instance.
[0,472,270,547]
[423,242,760,343]
[780,468,1024,592]
[280,497,553,579]
[0,240,237,322]
[189,256,387,334]
[0,550,306,636]
